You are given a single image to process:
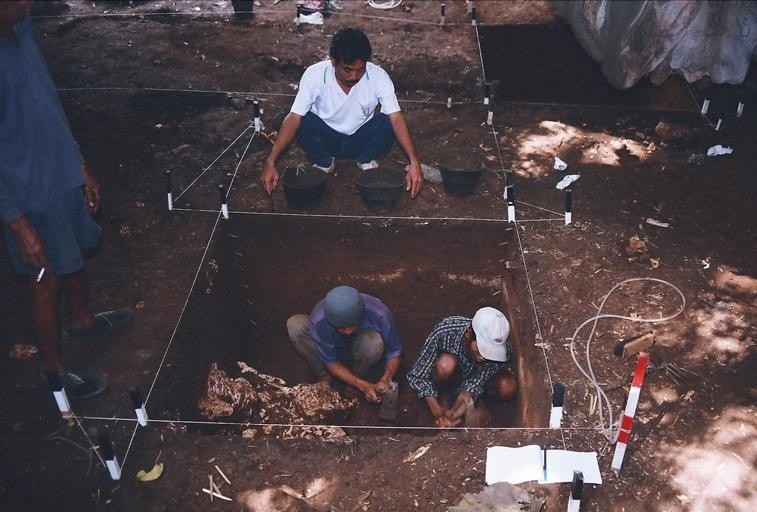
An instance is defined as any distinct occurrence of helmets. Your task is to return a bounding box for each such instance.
[323,284,364,329]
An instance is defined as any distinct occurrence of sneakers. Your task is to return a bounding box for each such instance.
[70,306,133,345]
[38,372,108,400]
[312,157,336,174]
[356,159,379,170]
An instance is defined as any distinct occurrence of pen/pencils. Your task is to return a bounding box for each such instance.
[201,462,236,504]
[543,444,547,478]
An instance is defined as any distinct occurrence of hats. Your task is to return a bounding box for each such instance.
[472,306,511,363]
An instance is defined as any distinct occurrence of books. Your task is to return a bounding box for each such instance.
[485,444,605,487]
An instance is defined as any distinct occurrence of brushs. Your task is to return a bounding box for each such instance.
[614,329,655,359]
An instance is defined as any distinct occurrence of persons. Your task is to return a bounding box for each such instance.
[260,28,424,199]
[286,285,403,402]
[405,305,518,433]
[1,1,134,401]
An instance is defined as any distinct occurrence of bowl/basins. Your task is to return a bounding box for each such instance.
[356,168,405,211]
[281,165,327,209]
[439,155,485,197]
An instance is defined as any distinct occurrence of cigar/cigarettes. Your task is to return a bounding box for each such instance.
[36,267,45,282]
